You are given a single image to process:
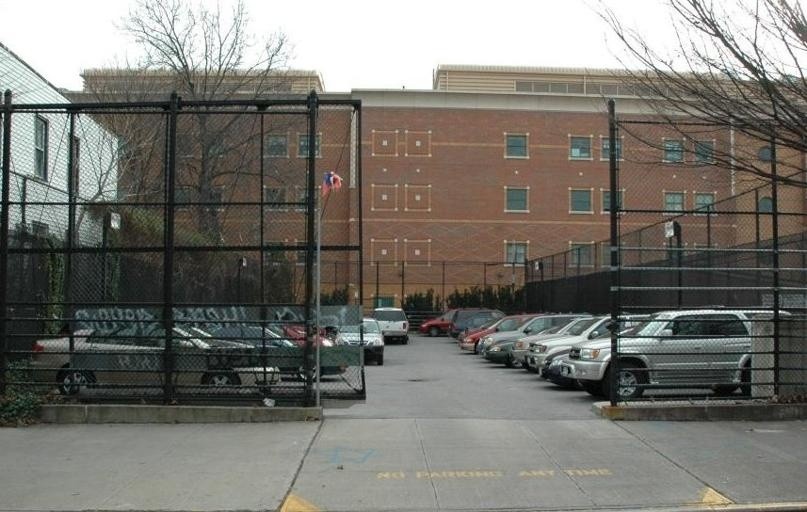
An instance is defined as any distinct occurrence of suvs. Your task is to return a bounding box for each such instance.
[372,307,409,344]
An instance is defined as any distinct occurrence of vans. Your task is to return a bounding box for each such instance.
[448,306,508,336]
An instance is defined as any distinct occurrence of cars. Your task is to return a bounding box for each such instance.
[421,307,488,337]
[336,317,383,367]
